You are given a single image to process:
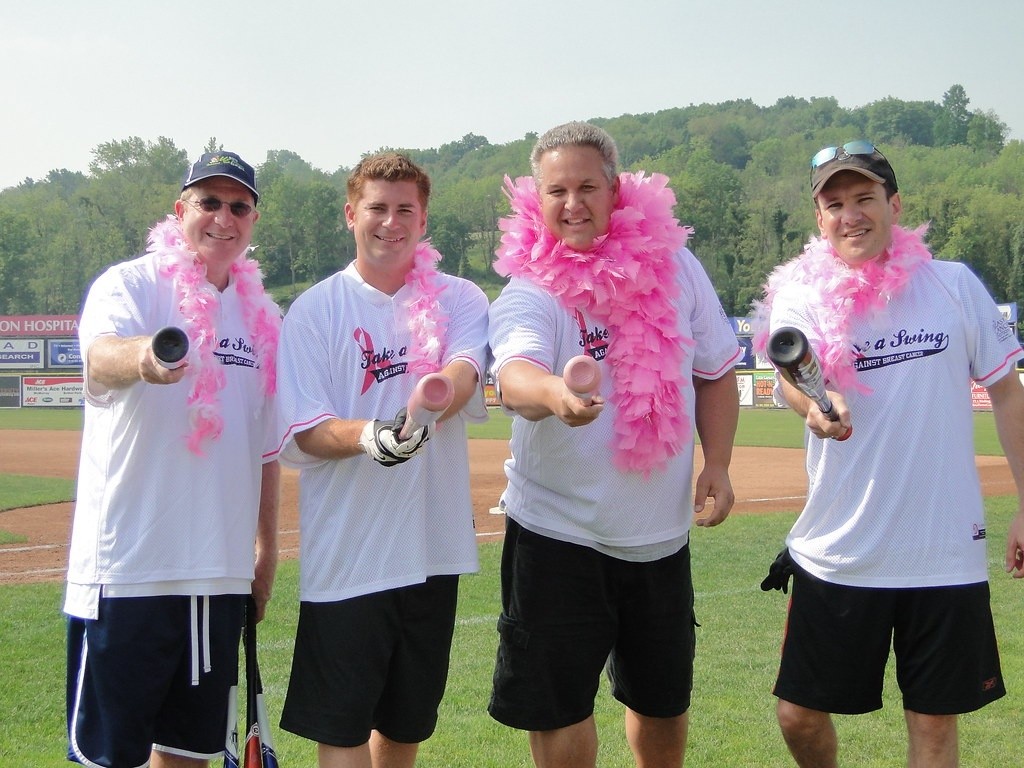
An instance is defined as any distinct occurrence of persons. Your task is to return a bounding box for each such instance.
[487,119,742,768]
[273,151,492,768]
[767,140,1024,768]
[62,150,281,768]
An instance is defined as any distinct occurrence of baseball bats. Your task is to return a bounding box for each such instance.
[399,372,455,442]
[765,327,853,441]
[243,596,279,768]
[223,657,240,767]
[563,355,602,406]
[152,326,191,370]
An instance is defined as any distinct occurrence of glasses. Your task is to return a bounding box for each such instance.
[187,197,257,219]
[810,140,896,188]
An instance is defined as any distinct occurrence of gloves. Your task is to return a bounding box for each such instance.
[392,407,436,454]
[358,418,411,467]
[760,549,794,594]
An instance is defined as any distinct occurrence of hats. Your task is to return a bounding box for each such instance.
[810,147,897,203]
[181,151,259,207]
